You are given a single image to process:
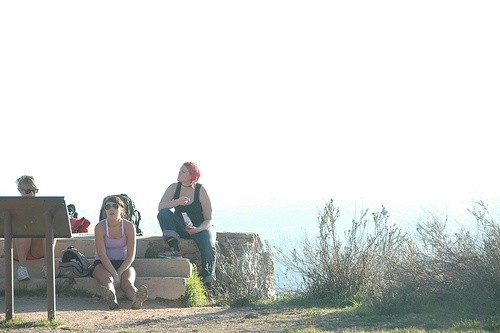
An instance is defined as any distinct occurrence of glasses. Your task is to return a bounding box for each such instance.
[22,189,39,194]
[105,204,120,210]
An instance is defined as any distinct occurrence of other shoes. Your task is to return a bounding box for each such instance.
[158,247,182,259]
[131,286,148,309]
[16,265,30,281]
[41,264,48,278]
[104,289,119,310]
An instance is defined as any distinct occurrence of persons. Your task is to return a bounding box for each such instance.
[157,161,217,291]
[89,195,149,310]
[3,175,58,281]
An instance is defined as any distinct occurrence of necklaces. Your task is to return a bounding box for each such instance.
[182,182,193,188]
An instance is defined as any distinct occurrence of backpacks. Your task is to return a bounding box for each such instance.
[99,194,143,236]
[56,246,92,278]
